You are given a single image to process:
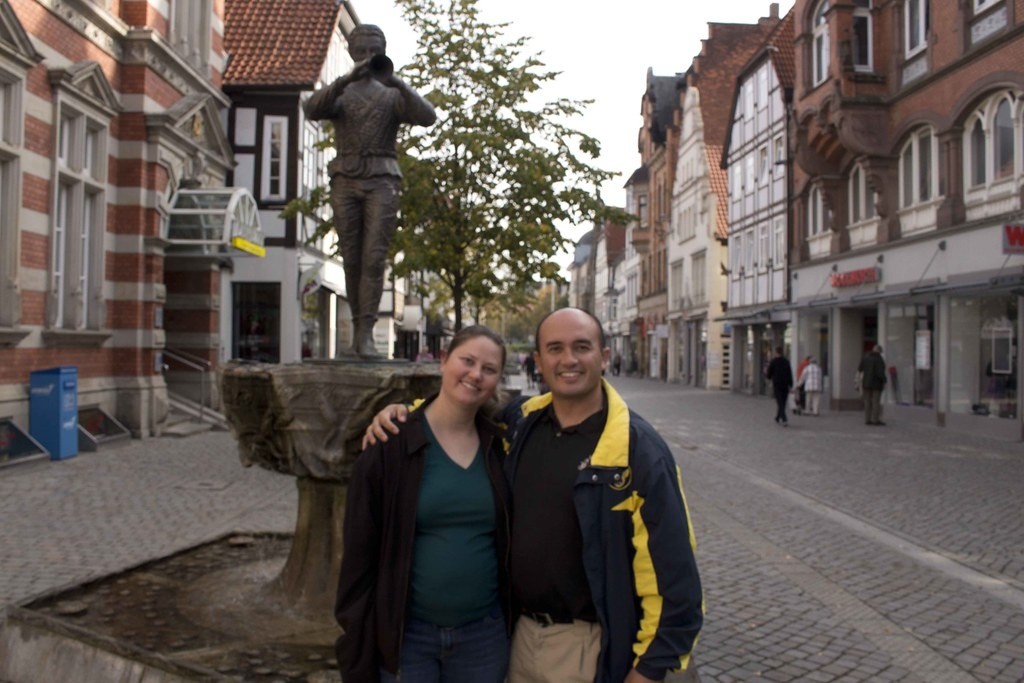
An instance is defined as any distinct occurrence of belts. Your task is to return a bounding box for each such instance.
[522,607,553,627]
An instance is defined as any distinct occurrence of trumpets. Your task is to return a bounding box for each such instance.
[363,52,396,86]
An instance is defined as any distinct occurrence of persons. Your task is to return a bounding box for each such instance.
[611,352,621,376]
[794,358,823,415]
[359,306,711,683]
[766,345,793,426]
[301,23,435,360]
[333,324,515,683]
[520,351,536,390]
[794,355,812,412]
[853,345,887,426]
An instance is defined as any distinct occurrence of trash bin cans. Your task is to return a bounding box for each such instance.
[29,366,79,461]
